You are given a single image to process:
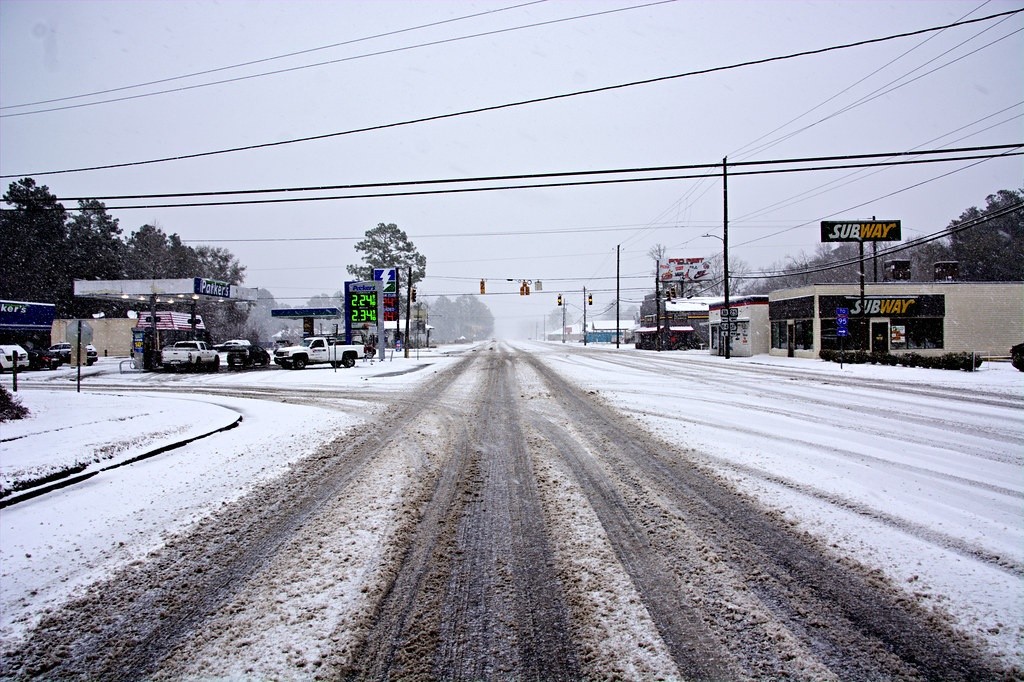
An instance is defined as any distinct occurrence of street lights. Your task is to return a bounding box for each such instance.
[702,232,733,359]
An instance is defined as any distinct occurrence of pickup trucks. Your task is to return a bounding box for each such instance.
[162,340,221,372]
[273,336,367,371]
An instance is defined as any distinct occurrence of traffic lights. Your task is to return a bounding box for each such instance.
[558,295,562,306]
[480,280,486,294]
[519,287,525,295]
[410,288,417,302]
[526,287,531,295]
[523,282,527,287]
[588,294,593,306]
[416,321,422,331]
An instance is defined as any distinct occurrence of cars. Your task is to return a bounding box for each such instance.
[227,345,271,371]
[329,340,376,358]
[212,339,252,352]
[18,339,66,372]
[0,335,31,373]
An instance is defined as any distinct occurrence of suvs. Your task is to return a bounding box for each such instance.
[46,342,99,367]
